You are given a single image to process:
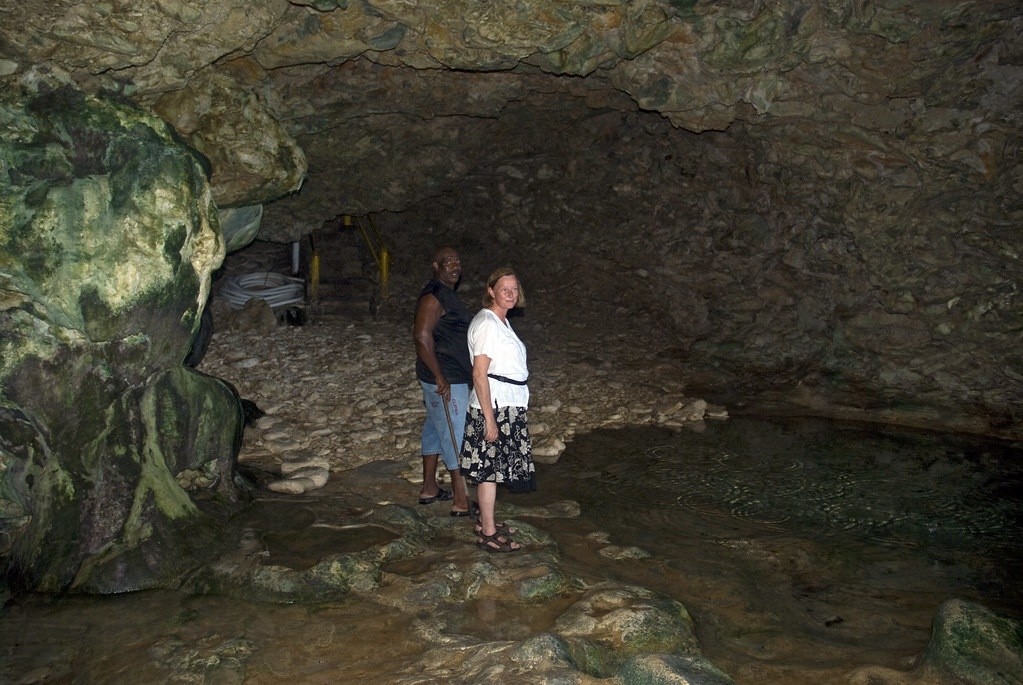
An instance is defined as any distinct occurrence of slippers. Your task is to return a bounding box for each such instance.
[419,489,454,504]
[450,500,480,517]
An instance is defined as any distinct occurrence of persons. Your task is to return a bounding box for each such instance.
[414,247,479,516]
[459,268,536,552]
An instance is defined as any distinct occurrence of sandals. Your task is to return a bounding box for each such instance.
[473,517,519,536]
[477,529,521,553]
